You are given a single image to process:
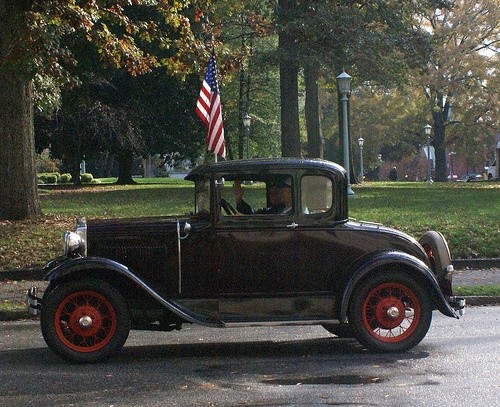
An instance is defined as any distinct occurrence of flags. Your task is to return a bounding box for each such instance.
[195,45,226,160]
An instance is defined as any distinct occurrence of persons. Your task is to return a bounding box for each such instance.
[231,177,308,216]
[389,166,398,182]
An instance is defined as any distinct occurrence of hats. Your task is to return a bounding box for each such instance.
[277,177,293,189]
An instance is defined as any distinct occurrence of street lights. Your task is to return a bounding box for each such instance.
[242,113,252,158]
[336,67,355,195]
[357,136,365,183]
[27,158,465,365]
[423,123,436,184]
